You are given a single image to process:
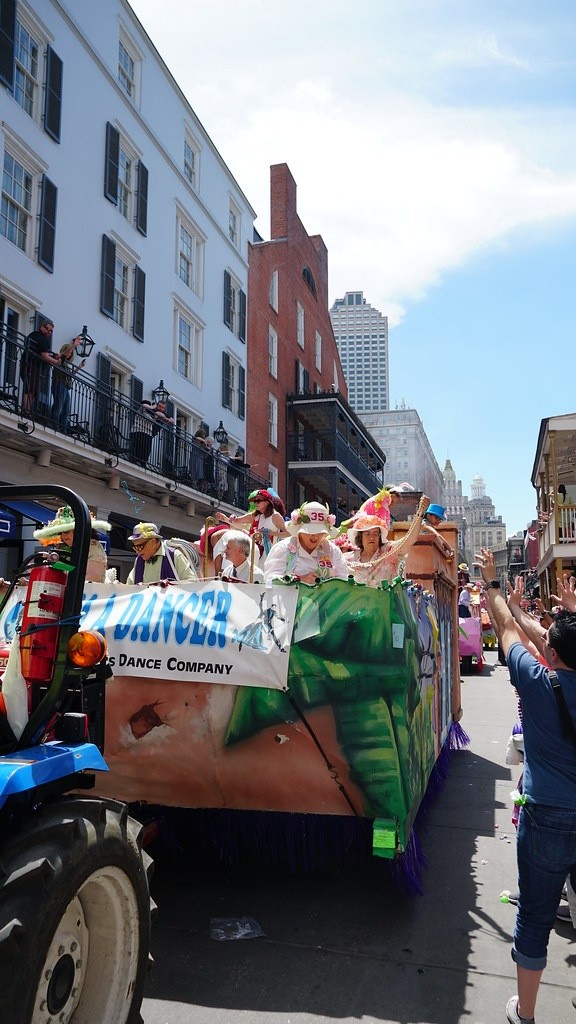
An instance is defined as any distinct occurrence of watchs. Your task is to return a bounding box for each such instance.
[486,581,499,591]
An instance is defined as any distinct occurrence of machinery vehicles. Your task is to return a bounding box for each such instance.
[0,480,158,1024]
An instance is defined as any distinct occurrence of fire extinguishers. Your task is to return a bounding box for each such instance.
[0,541,69,683]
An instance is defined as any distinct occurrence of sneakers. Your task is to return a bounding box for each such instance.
[555,906,573,922]
[507,892,521,905]
[506,994,534,1024]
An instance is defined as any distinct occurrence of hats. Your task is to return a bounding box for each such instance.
[165,538,199,569]
[284,502,340,538]
[424,504,447,521]
[33,505,110,540]
[464,583,474,589]
[127,522,162,539]
[248,490,272,501]
[200,524,229,553]
[334,489,393,550]
[460,563,469,571]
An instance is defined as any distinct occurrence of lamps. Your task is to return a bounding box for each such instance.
[37,449,52,467]
[160,495,169,507]
[213,420,228,442]
[152,380,171,405]
[186,502,195,517]
[72,325,95,358]
[110,476,121,490]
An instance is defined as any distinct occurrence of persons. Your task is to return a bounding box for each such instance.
[32,506,111,583]
[126,522,199,584]
[48,334,87,433]
[473,548,576,1023]
[200,525,264,583]
[230,488,292,570]
[189,428,207,491]
[20,318,61,419]
[458,564,488,620]
[215,444,244,501]
[264,501,350,580]
[548,484,574,542]
[528,506,554,536]
[227,452,259,505]
[203,435,216,493]
[335,483,454,587]
[130,400,175,467]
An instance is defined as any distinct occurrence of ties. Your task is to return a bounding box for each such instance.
[233,568,237,578]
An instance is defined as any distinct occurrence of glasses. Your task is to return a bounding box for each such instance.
[132,538,151,552]
[57,529,70,536]
[540,632,552,647]
[43,325,54,333]
[253,499,266,504]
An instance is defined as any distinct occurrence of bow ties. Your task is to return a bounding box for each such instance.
[147,555,159,563]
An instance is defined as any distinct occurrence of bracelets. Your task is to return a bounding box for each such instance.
[541,611,546,616]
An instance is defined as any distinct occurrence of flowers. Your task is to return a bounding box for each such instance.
[327,513,337,528]
[290,503,310,525]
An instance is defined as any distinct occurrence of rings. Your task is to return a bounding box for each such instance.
[516,588,519,593]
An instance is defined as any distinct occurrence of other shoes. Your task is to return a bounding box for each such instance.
[561,887,567,901]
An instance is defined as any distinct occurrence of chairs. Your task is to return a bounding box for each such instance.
[100,424,135,460]
[0,383,19,412]
[67,414,90,443]
[165,454,191,484]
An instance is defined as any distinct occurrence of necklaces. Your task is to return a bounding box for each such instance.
[348,504,424,565]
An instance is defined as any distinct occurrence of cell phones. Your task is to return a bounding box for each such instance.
[59,355,66,365]
